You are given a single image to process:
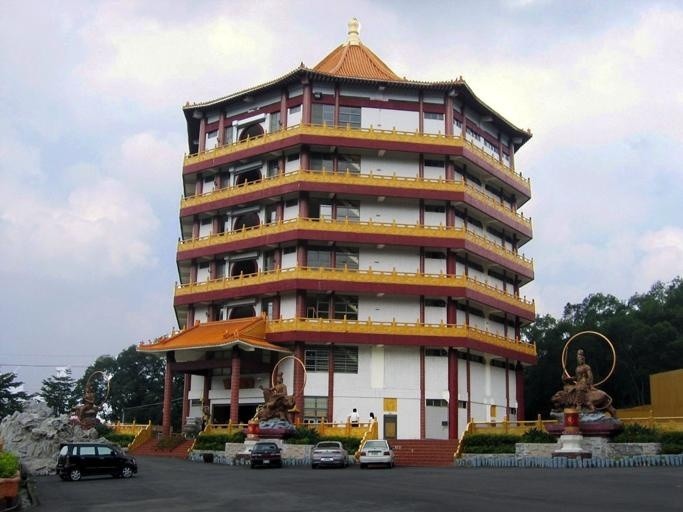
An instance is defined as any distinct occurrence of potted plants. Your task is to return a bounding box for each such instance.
[0,448,22,498]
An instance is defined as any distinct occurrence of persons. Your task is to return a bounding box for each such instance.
[200,392,210,427]
[73,385,96,420]
[350,408,358,427]
[368,412,374,422]
[553,347,615,413]
[258,370,296,422]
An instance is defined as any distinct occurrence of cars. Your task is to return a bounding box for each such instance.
[358,439,395,470]
[310,440,350,470]
[248,441,283,469]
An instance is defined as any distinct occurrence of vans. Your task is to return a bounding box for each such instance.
[55,440,138,483]
